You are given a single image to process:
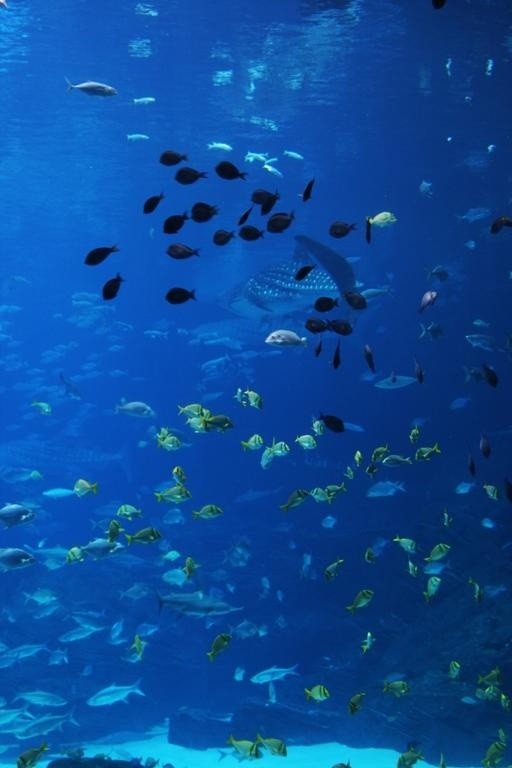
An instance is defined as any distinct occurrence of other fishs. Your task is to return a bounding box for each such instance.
[0,0,509,768]
